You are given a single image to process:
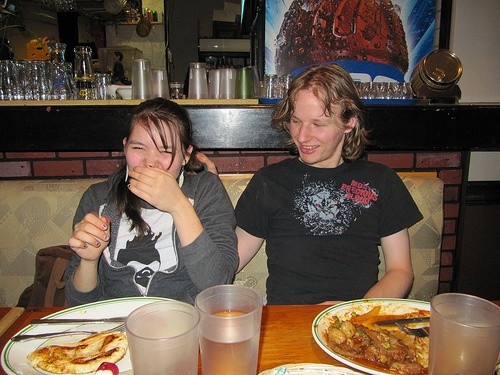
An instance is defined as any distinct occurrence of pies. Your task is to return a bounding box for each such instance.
[26,330,128,375]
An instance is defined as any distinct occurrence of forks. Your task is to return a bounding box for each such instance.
[394,321,430,337]
[11,322,125,343]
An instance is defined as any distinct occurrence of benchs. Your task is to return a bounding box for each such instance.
[0,174,444,307]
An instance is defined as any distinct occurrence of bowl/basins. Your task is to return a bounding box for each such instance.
[116,87,132,98]
[108,85,121,98]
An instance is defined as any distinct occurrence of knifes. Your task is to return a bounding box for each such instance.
[372,316,430,326]
[30,316,128,322]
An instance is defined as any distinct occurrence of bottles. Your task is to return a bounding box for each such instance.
[72,46,95,99]
[48,43,66,60]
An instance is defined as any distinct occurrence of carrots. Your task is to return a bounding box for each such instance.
[352,306,405,323]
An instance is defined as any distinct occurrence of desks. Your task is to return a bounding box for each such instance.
[0,301,500,375]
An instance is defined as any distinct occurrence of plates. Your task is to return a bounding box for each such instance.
[0,297,193,375]
[257,362,366,375]
[312,297,431,375]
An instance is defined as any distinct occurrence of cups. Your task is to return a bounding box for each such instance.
[427,293,500,375]
[279,74,293,98]
[125,300,202,375]
[355,80,411,100]
[188,62,209,101]
[194,284,263,375]
[95,74,110,99]
[170,82,186,101]
[209,67,260,100]
[260,74,280,99]
[150,69,169,99]
[133,58,150,102]
[0,58,72,101]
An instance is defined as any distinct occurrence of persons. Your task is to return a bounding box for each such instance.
[195,63,424,305]
[64,97,240,305]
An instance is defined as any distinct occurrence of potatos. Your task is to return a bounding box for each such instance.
[328,321,356,344]
